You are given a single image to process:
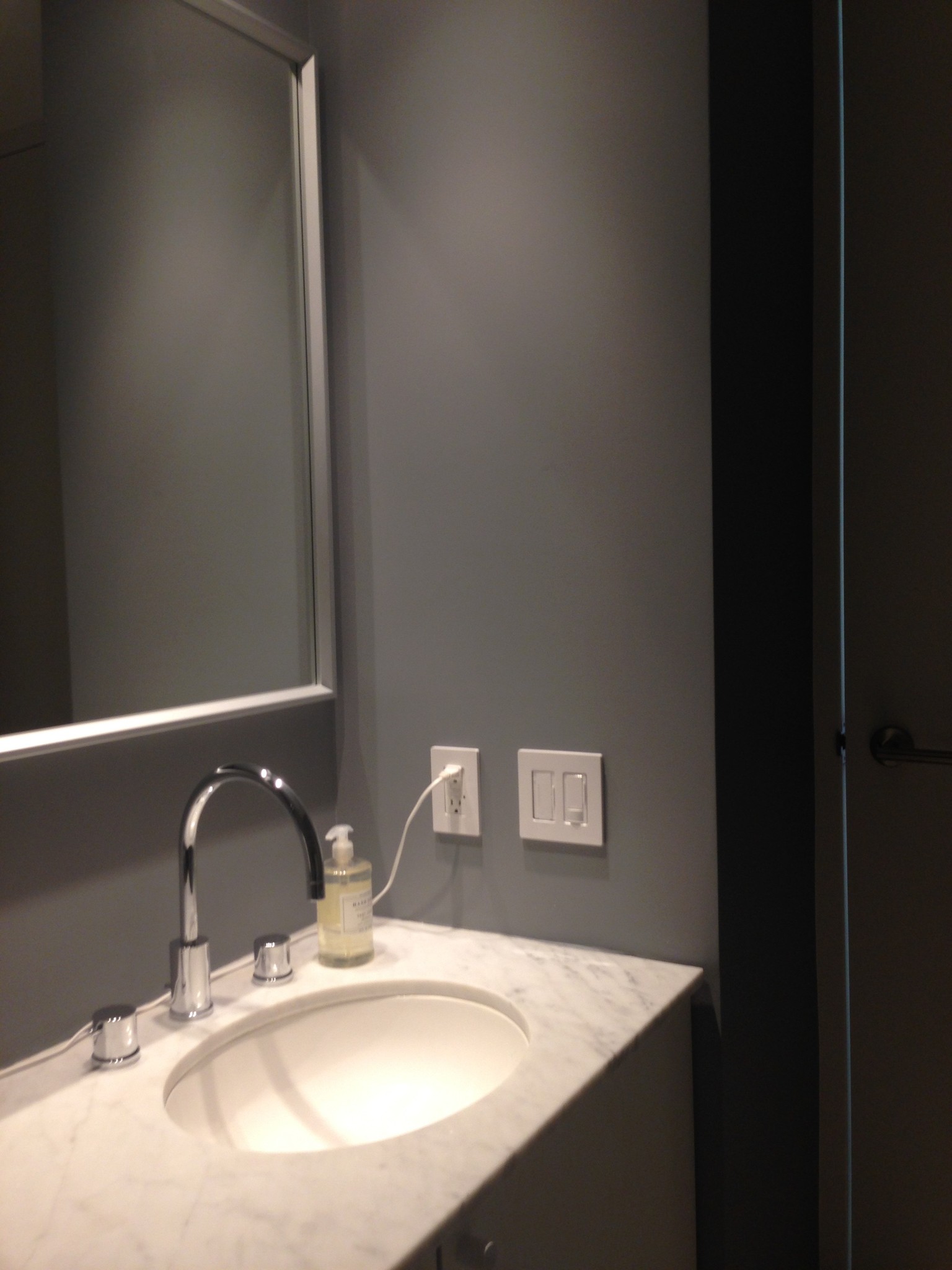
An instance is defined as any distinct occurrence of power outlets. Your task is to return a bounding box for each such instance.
[430,746,481,836]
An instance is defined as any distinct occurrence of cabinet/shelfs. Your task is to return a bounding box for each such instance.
[409,988,697,1270]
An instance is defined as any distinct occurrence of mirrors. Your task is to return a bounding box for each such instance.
[0,0,337,761]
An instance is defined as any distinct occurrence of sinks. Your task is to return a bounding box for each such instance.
[157,976,532,1159]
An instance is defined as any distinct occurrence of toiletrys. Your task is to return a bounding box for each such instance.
[314,823,377,972]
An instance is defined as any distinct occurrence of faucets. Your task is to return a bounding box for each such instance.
[166,763,328,1020]
